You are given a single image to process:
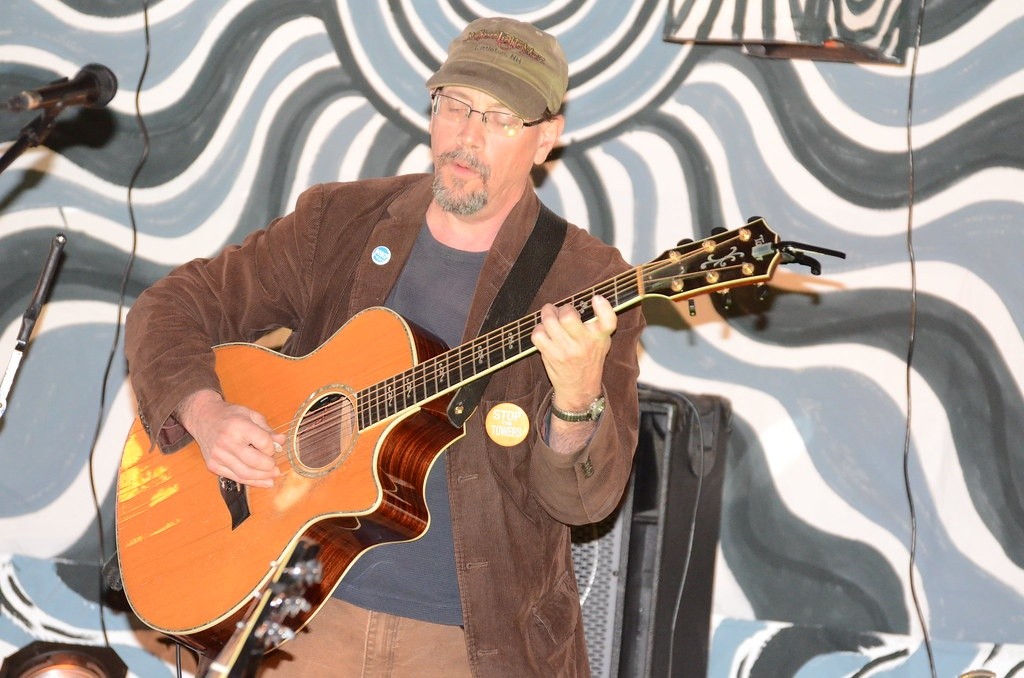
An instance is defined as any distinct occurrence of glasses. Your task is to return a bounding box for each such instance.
[430,88,548,138]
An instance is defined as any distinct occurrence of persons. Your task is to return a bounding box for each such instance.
[126,17,645,678]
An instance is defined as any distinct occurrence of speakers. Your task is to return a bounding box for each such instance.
[571,381,731,678]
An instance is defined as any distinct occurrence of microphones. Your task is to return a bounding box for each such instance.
[5,62,116,115]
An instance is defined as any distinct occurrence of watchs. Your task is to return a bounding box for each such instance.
[551,388,605,422]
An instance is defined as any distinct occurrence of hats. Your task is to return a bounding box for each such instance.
[425,15,569,124]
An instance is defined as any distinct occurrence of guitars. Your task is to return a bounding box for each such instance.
[110,210,848,661]
